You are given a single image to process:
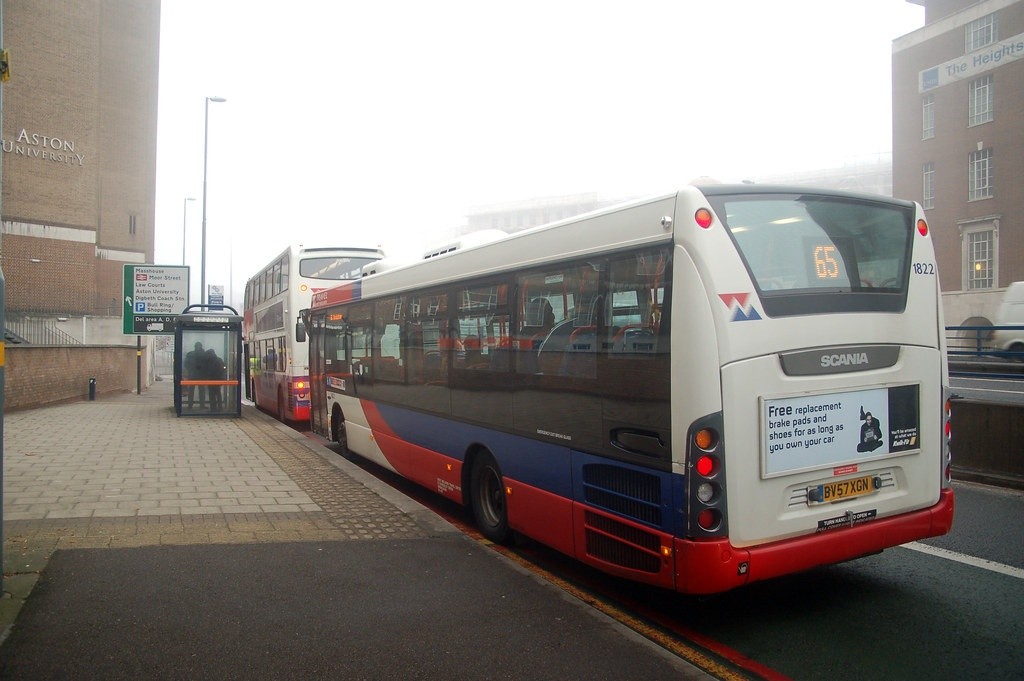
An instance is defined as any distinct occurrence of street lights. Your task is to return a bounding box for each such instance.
[199,95,229,313]
[180,197,196,263]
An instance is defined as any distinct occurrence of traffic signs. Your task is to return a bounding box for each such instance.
[122,262,190,336]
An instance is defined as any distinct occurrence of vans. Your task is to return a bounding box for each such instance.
[989,280,1024,363]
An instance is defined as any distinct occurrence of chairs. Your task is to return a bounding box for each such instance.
[352,315,659,384]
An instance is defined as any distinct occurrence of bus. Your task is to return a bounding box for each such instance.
[293,177,959,605]
[239,242,389,430]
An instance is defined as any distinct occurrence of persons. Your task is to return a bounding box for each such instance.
[250,352,260,375]
[529,298,555,328]
[263,349,277,370]
[856,405,883,453]
[184,342,225,411]
